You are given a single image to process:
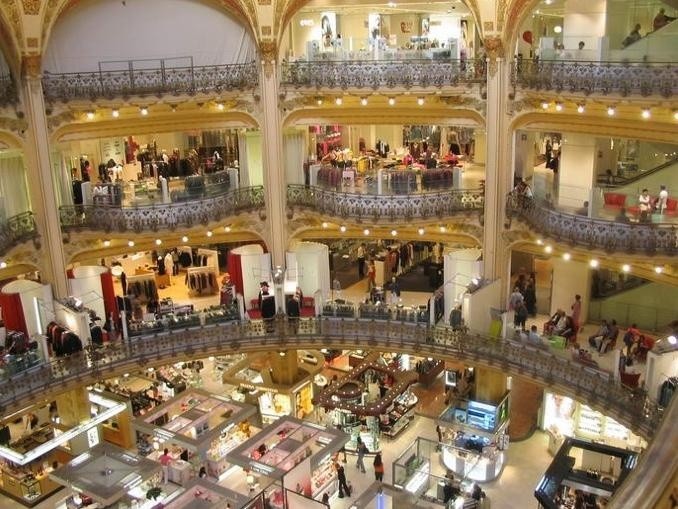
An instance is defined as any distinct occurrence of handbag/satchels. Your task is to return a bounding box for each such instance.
[364,448,368,453]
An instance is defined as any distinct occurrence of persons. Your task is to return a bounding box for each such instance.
[322,14,332,40]
[71,137,461,169]
[557,41,585,58]
[607,165,640,184]
[574,488,608,508]
[402,41,445,50]
[614,184,668,223]
[422,18,429,34]
[372,17,380,38]
[516,176,556,212]
[102,247,649,374]
[622,24,642,47]
[575,201,588,217]
[330,33,343,46]
[30,373,491,508]
[545,139,561,190]
[461,20,467,39]
[553,395,564,416]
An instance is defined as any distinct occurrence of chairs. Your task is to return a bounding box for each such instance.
[604,190,678,217]
[507,306,656,391]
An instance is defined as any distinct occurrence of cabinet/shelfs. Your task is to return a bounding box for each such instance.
[307,39,469,73]
